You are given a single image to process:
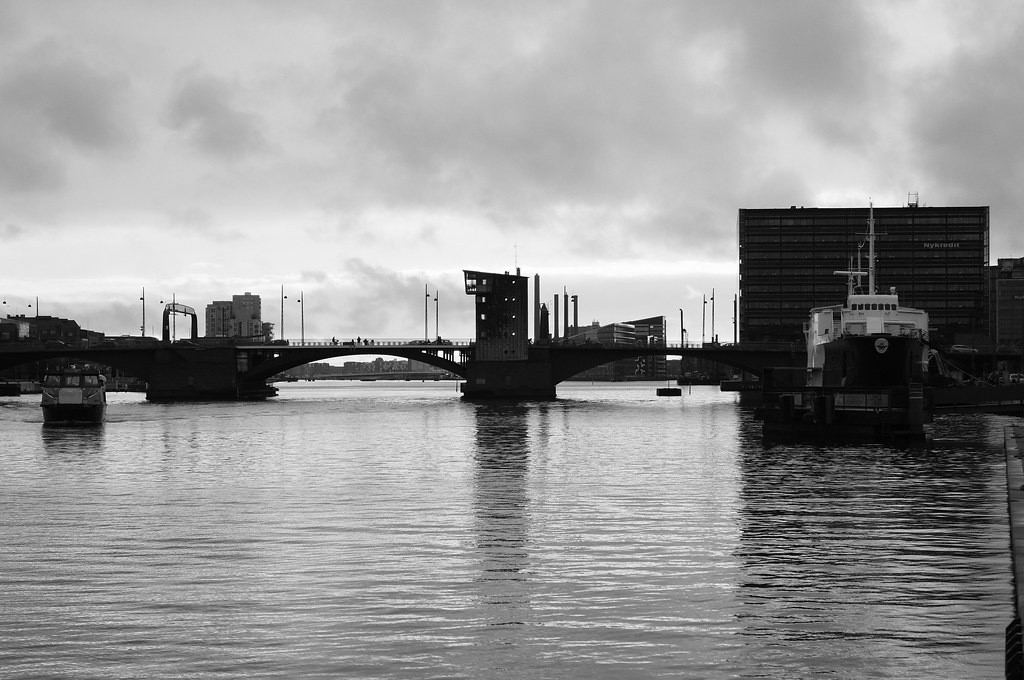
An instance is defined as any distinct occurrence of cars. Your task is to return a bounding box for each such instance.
[406,340,426,345]
[1009,374,1024,383]
[96,340,117,348]
[950,344,979,354]
[44,339,72,350]
[173,340,200,347]
[576,340,601,347]
[427,339,453,345]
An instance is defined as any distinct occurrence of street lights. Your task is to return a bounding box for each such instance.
[298,293,304,345]
[140,287,145,338]
[281,285,288,339]
[425,283,430,341]
[703,294,708,342]
[711,289,715,336]
[434,290,439,338]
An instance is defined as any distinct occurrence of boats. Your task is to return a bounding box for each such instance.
[40,364,108,427]
[761,204,931,445]
